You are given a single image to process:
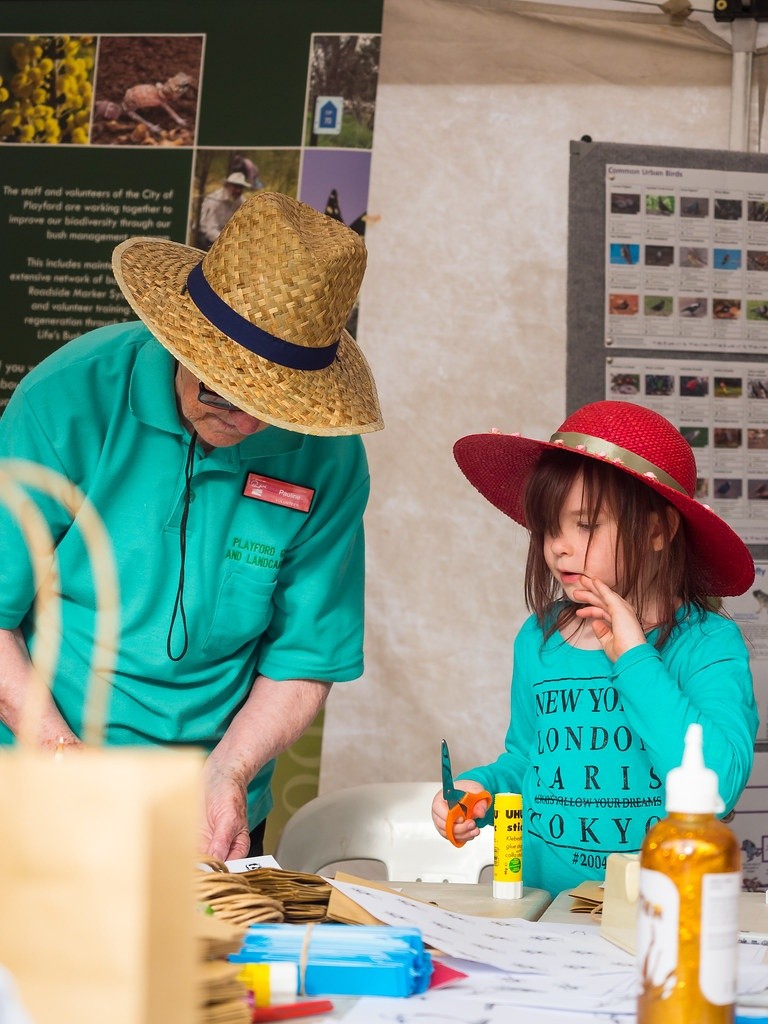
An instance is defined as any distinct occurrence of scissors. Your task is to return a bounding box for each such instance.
[441,738,493,848]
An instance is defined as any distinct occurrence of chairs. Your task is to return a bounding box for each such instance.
[277,781,494,884]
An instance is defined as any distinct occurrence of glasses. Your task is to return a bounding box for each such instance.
[197,377,243,411]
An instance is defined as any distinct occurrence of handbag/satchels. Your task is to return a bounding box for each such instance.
[0,457,208,1024]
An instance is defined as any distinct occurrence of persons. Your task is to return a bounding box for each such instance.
[200,156,263,254]
[430,398,757,899]
[0,194,383,869]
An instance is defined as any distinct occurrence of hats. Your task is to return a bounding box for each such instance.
[452,401,755,598]
[222,172,252,188]
[111,190,385,437]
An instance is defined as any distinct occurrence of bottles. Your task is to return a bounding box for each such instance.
[637,721,745,1024]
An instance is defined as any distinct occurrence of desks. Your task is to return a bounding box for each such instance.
[377,882,766,1024]
